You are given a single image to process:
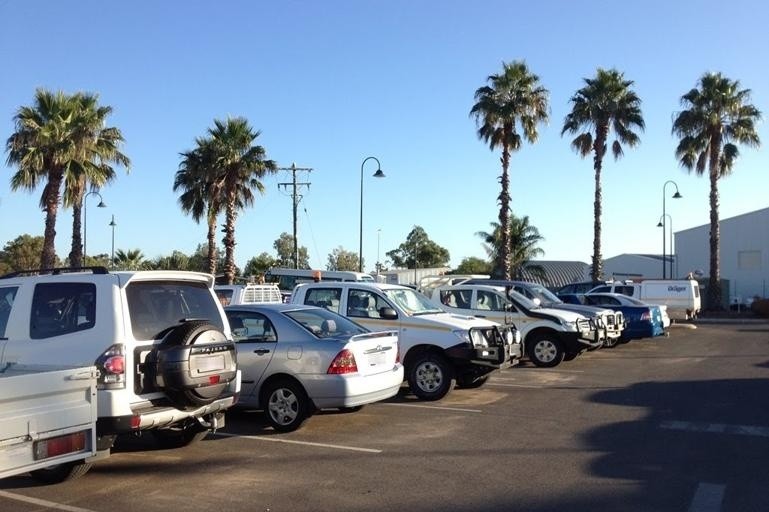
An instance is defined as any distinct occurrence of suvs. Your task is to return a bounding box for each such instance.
[1,266,244,481]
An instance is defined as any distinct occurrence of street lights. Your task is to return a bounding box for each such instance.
[658,215,672,280]
[662,180,682,280]
[83,192,106,272]
[359,156,384,273]
[109,215,115,269]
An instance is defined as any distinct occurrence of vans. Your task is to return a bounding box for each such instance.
[585,278,703,322]
[265,267,375,308]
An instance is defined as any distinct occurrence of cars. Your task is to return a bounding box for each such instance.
[223,304,405,432]
[418,279,670,368]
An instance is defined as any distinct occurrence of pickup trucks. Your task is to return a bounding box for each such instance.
[285,282,525,403]
[211,282,285,306]
[0,363,98,482]
